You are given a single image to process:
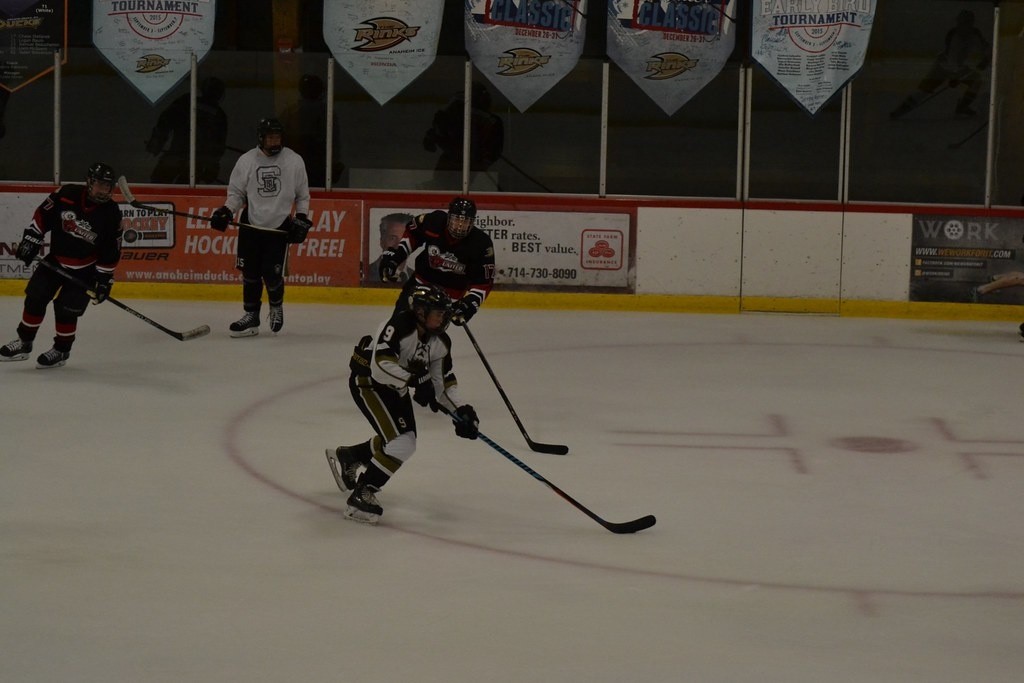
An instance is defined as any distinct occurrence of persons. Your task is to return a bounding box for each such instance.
[424,84,504,190]
[890,9,992,120]
[277,73,344,187]
[325,285,480,526]
[368,213,414,286]
[0,164,125,370]
[145,77,227,184]
[210,119,313,338]
[379,198,495,395]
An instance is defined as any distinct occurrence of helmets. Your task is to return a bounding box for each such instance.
[257,116,284,154]
[298,72,329,102]
[408,282,453,315]
[199,76,224,102]
[86,163,115,203]
[445,198,477,240]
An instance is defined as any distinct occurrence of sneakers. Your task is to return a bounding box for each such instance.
[229,300,263,337]
[324,439,376,492]
[35,342,72,369]
[0,326,35,361]
[343,471,383,522]
[266,300,284,333]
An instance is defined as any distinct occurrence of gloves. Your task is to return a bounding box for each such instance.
[452,404,480,440]
[405,365,437,415]
[15,228,46,267]
[451,293,481,326]
[286,216,314,243]
[86,273,115,306]
[379,251,400,284]
[210,206,233,232]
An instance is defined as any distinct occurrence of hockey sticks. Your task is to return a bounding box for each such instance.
[32,256,211,343]
[118,176,290,235]
[433,401,656,535]
[458,315,570,456]
